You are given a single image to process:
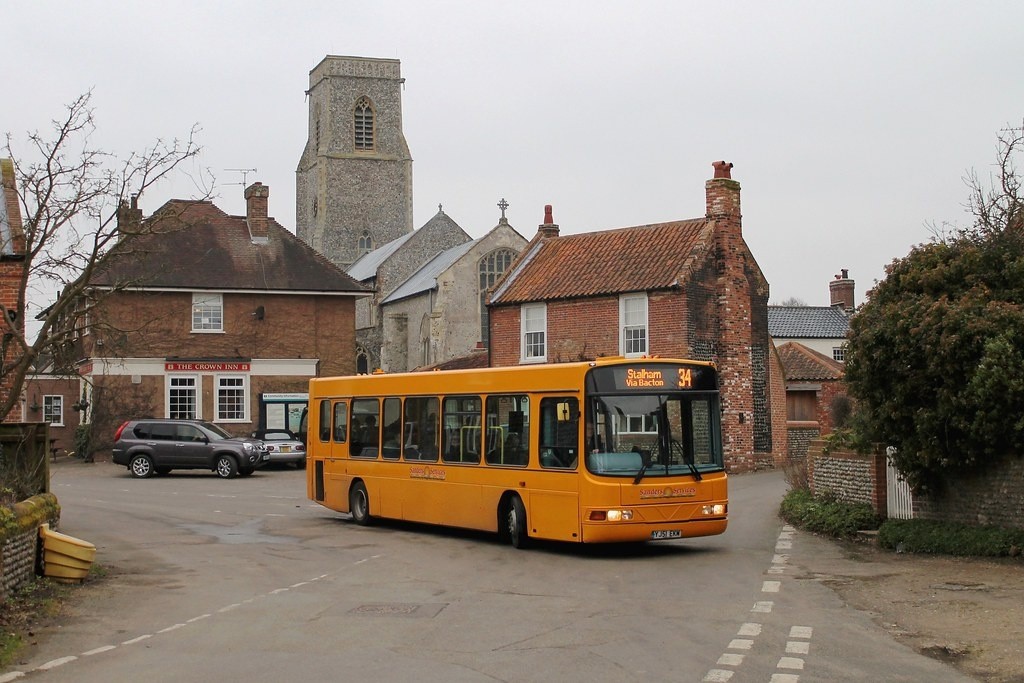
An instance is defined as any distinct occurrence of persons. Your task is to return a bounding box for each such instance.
[346,412,481,463]
[486,432,520,464]
[567,420,599,467]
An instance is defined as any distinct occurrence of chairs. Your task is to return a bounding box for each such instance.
[336,421,496,466]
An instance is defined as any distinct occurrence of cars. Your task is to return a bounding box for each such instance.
[451,430,480,454]
[252,429,306,463]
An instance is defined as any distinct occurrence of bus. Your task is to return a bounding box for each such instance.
[306,355,729,547]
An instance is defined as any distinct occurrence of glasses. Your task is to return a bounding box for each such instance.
[587,427,593,429]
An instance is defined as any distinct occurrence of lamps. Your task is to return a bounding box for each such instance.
[29,393,41,412]
[251,306,264,320]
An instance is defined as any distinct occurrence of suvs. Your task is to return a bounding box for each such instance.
[111,419,270,479]
